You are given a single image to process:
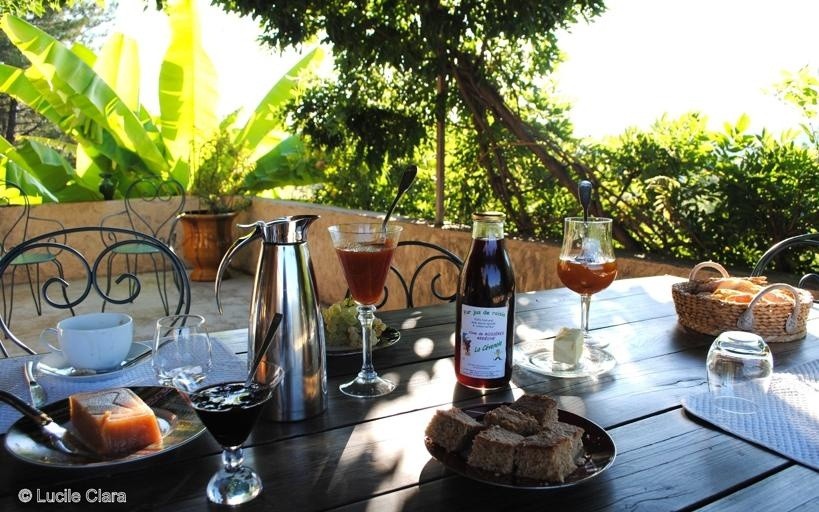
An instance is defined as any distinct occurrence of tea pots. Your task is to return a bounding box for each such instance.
[214,214,328,422]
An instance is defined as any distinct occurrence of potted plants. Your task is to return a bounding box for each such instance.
[175,126,257,282]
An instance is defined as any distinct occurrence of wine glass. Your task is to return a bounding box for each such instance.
[327,222,403,400]
[555,214,617,351]
[172,358,285,507]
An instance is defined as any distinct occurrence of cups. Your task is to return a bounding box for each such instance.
[151,313,214,387]
[41,311,136,371]
[705,330,773,414]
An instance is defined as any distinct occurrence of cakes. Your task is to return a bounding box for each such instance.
[469,425,523,476]
[538,420,584,459]
[516,430,578,482]
[509,395,558,430]
[485,404,538,442]
[424,405,483,453]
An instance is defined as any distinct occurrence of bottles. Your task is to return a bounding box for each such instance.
[453,210,516,393]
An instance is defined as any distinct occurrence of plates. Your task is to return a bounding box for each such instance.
[512,339,618,379]
[328,327,401,358]
[4,388,207,468]
[29,341,154,383]
[424,401,618,491]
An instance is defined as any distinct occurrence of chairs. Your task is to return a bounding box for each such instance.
[0,227,192,358]
[102,178,185,315]
[751,232,819,302]
[0,177,76,340]
[343,240,465,315]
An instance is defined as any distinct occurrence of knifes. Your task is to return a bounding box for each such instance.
[1,389,96,457]
[23,359,49,410]
